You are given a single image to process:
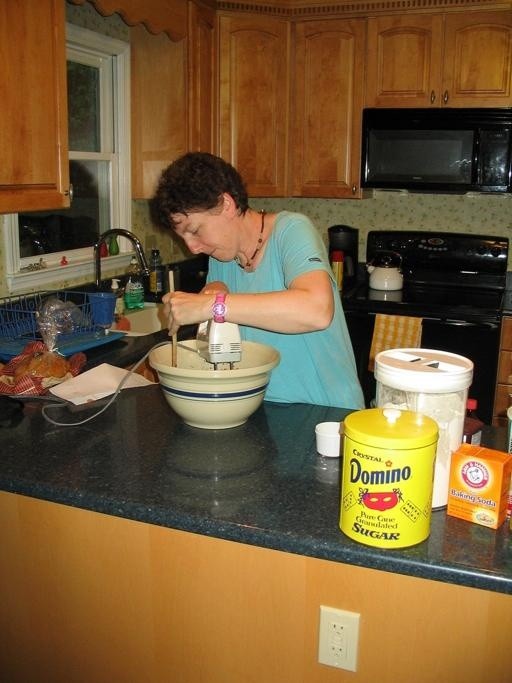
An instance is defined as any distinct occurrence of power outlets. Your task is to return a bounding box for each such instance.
[318,605,361,673]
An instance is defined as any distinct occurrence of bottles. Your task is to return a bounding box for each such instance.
[462,398,484,446]
[125,256,145,310]
[331,250,343,292]
[149,250,163,297]
[341,409,439,550]
[373,348,473,512]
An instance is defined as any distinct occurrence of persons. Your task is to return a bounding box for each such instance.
[149,152,365,413]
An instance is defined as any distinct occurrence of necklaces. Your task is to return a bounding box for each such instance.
[234,206,265,271]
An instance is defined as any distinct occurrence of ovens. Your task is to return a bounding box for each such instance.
[340,297,501,419]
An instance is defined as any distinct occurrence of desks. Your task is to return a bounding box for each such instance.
[0,385,510,683]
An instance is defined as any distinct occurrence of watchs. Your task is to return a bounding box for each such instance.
[211,291,227,326]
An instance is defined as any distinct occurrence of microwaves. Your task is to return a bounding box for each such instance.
[361,109,511,192]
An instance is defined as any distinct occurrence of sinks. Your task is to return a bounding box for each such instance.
[106,301,171,337]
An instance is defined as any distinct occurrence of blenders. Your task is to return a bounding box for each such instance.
[328,226,360,286]
[205,295,245,373]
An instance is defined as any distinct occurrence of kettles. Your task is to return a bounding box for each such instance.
[365,250,404,292]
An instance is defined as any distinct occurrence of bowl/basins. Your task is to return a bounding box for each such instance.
[147,336,283,431]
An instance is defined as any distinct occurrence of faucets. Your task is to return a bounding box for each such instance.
[95,227,151,296]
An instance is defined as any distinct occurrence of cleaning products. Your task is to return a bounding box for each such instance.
[106,278,126,318]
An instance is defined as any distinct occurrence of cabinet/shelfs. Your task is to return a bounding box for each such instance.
[369,9,512,109]
[213,13,372,200]
[132,11,215,199]
[1,0,74,214]
